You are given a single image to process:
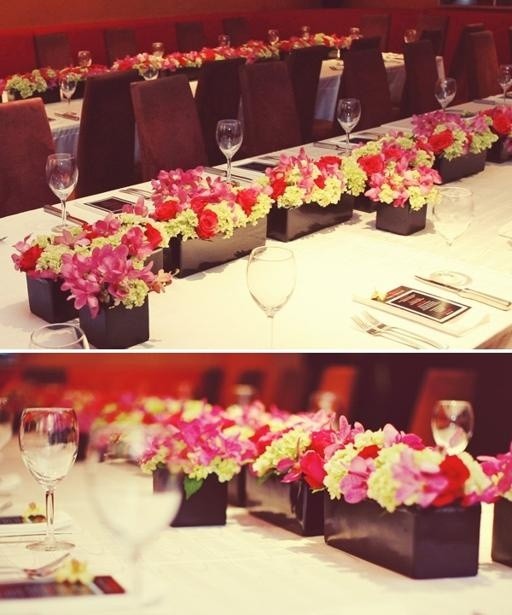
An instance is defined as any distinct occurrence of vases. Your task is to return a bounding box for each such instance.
[323,488,481,580]
[492,495,512,568]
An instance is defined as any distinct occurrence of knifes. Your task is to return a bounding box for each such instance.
[44,204,87,225]
[312,141,352,151]
[205,167,253,181]
[414,274,511,312]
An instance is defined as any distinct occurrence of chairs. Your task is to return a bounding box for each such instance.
[197,362,477,450]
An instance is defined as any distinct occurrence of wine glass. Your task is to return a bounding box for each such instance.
[497,63,512,108]
[59,75,78,116]
[45,152,80,233]
[336,97,362,158]
[215,118,244,189]
[29,323,91,349]
[402,29,417,44]
[77,50,91,68]
[430,186,473,287]
[434,76,458,113]
[151,41,165,60]
[0,396,15,453]
[245,245,297,349]
[428,397,476,458]
[349,27,360,42]
[267,29,280,45]
[142,65,160,82]
[300,25,312,40]
[217,34,231,51]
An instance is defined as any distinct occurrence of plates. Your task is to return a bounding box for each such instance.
[0,506,76,533]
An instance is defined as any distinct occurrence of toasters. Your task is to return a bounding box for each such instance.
[20,406,81,551]
[85,425,184,611]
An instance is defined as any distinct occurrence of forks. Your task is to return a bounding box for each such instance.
[362,310,448,350]
[352,314,423,350]
[0,552,75,582]
